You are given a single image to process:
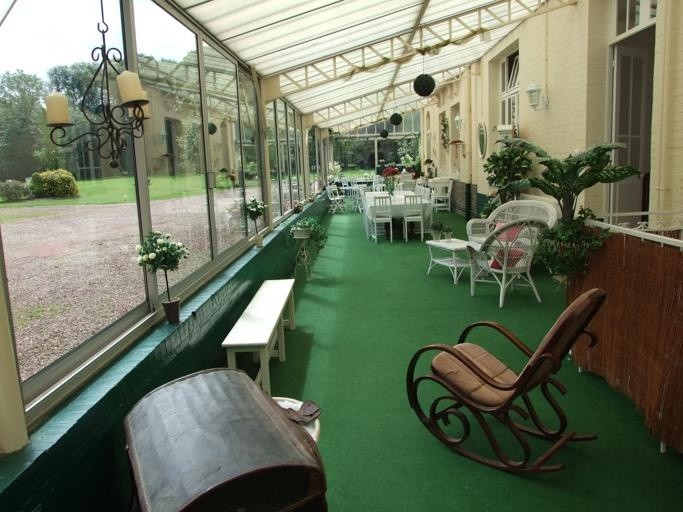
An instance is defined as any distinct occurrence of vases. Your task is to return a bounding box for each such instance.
[255,235,264,248]
[161,299,180,322]
[309,199,314,202]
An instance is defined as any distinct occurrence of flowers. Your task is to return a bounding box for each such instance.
[382,166,400,177]
[326,161,343,176]
[307,191,316,198]
[292,200,303,208]
[135,231,190,296]
[246,194,268,234]
[440,118,450,151]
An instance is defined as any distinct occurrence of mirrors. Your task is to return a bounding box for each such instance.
[477,120,487,159]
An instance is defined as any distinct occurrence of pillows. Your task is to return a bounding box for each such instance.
[496,223,521,241]
[491,249,524,268]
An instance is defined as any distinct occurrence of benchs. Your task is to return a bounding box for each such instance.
[221,278,296,397]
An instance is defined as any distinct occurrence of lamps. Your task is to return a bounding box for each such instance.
[45,0,150,168]
[454,111,462,130]
[525,80,548,111]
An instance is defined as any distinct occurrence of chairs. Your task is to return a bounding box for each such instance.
[407,287,607,471]
[466,199,557,279]
[465,218,550,308]
[326,176,453,243]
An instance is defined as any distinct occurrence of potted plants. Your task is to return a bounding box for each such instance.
[287,217,328,254]
